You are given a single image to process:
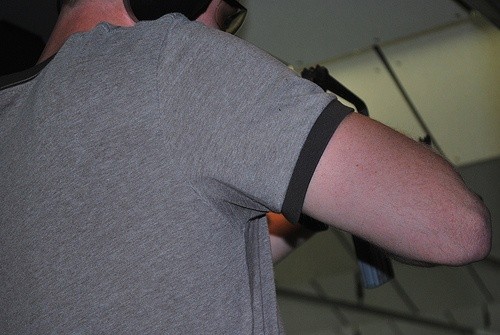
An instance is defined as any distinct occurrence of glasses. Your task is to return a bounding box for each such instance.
[224,1,247,33]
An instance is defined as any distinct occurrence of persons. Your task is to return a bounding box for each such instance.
[0,0,495,335]
